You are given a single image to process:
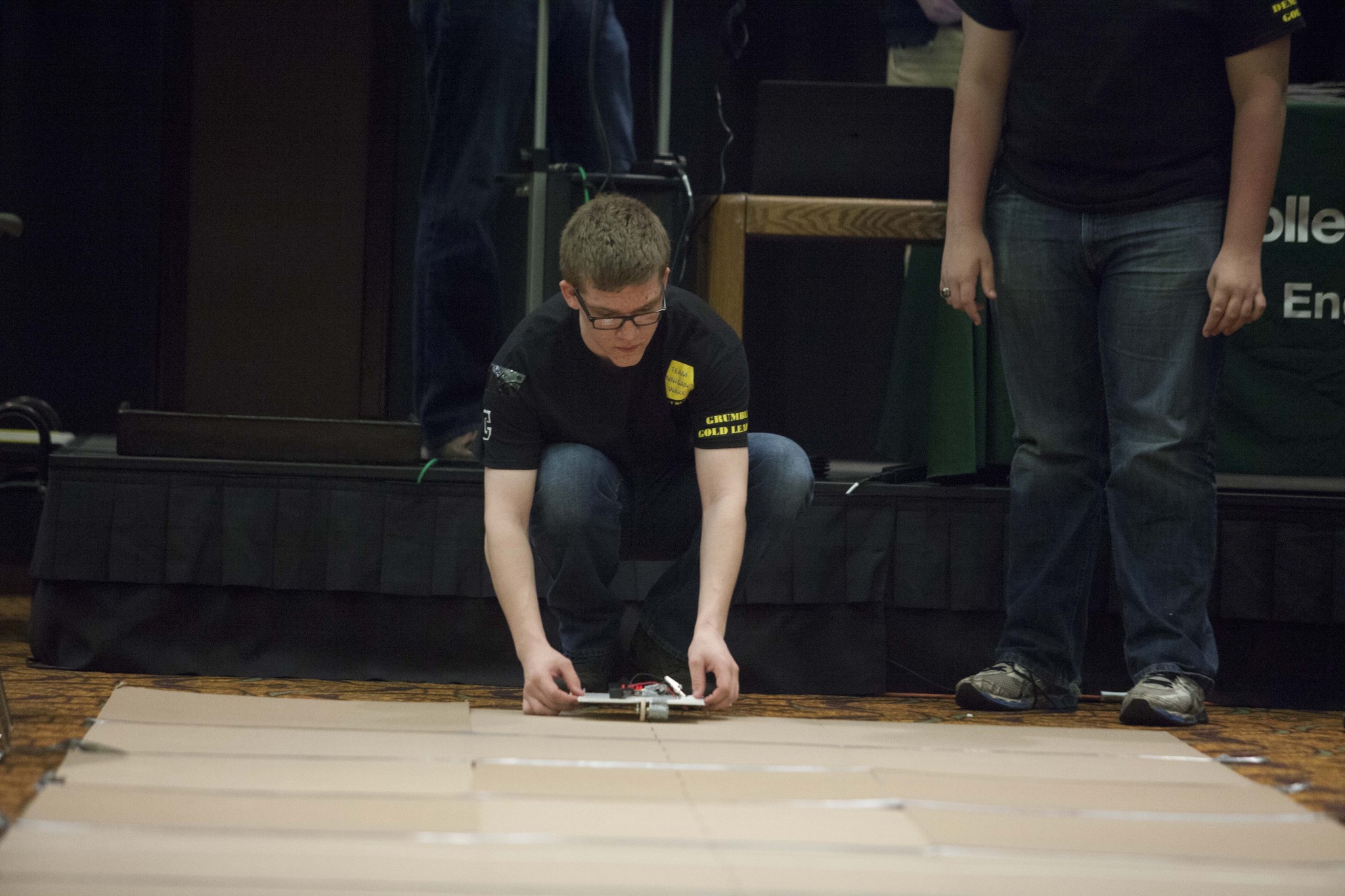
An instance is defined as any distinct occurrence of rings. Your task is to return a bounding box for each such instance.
[943,288,953,299]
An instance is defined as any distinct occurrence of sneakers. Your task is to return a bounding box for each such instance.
[1117,670,1209,726]
[954,654,1081,711]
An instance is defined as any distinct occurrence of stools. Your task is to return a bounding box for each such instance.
[693,190,985,478]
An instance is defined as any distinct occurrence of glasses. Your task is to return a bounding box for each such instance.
[570,282,668,330]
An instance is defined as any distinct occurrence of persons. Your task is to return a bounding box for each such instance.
[480,196,816,719]
[730,2,967,91]
[398,0,641,476]
[933,0,1305,726]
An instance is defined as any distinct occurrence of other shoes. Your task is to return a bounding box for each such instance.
[553,648,616,693]
[629,620,694,696]
[420,427,482,464]
